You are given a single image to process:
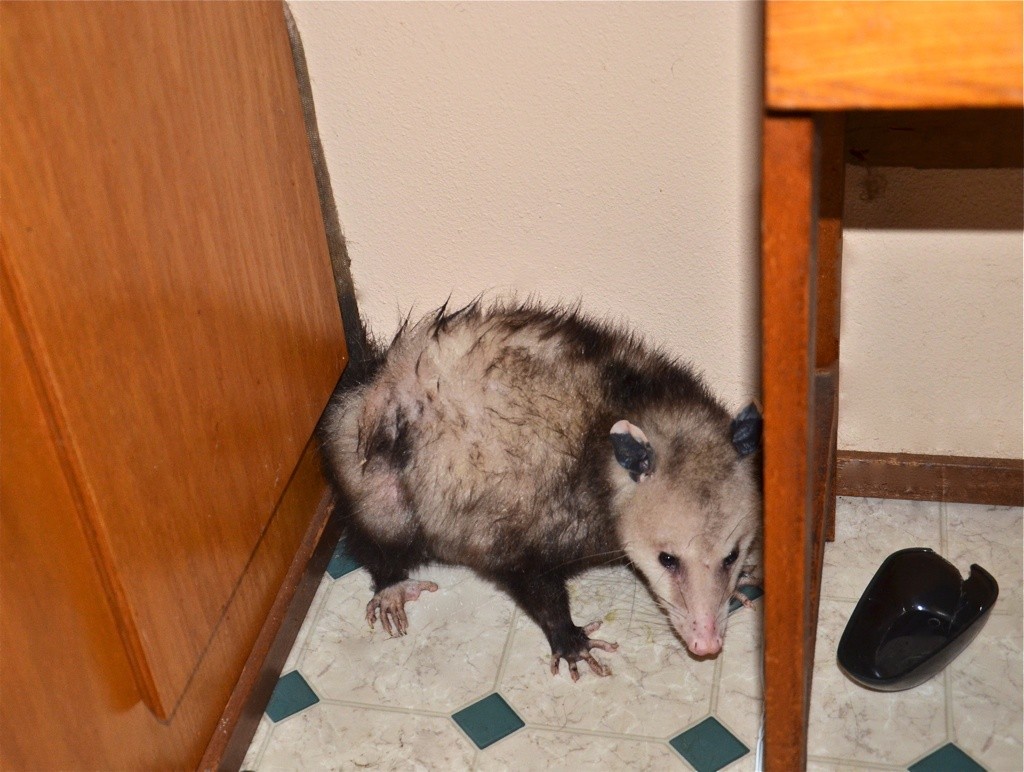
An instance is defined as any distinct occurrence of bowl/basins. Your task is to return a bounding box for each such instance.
[835,546,999,691]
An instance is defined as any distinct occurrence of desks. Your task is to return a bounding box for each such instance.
[762,0,1024,771]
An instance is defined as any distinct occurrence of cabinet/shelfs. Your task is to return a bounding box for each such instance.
[0,0,352,772]
